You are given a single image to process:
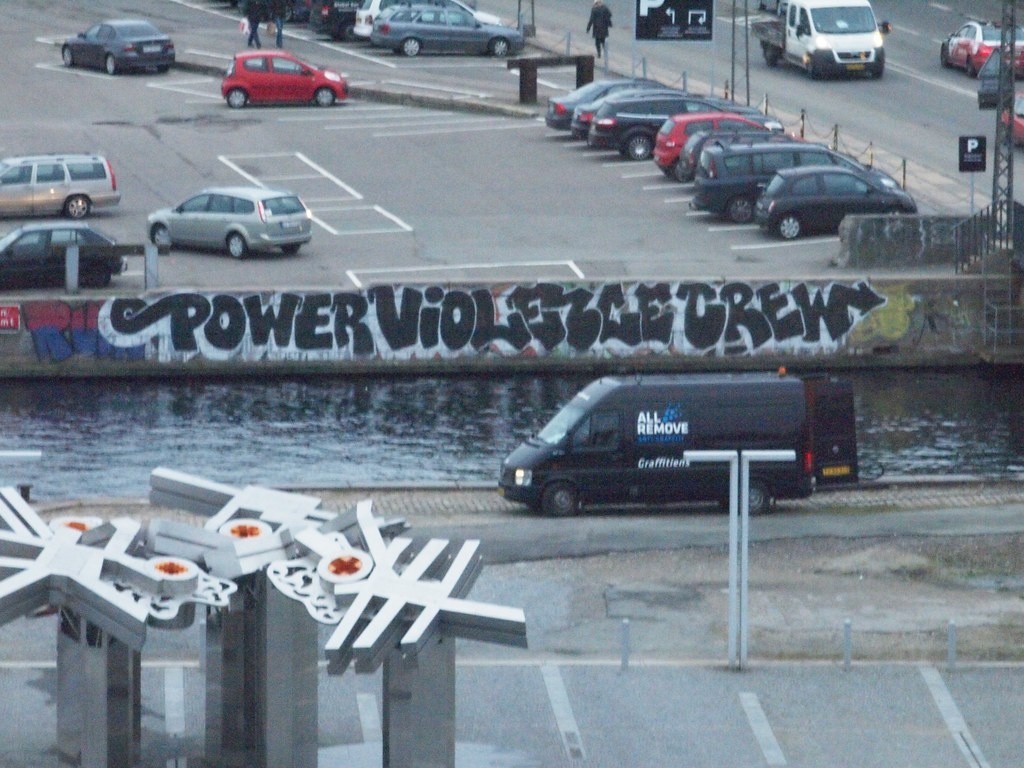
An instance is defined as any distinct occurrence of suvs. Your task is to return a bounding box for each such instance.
[1,221,130,287]
[309,0,374,42]
[589,97,784,162]
[369,0,524,58]
[148,187,314,260]
[353,0,503,38]
[1,151,122,220]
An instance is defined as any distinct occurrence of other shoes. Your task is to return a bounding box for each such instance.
[248,43,254,48]
[257,43,261,49]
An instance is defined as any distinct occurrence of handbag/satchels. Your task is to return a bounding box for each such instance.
[267,21,276,39]
[608,18,613,27]
[239,17,252,35]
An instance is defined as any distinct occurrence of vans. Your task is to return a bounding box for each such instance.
[688,139,903,223]
[497,366,861,519]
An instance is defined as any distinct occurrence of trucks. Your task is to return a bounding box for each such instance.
[750,0,891,80]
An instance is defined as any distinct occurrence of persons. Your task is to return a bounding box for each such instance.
[244,0,287,49]
[586,0,612,58]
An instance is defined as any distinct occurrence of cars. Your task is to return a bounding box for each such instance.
[676,131,806,182]
[756,165,917,240]
[546,78,694,131]
[63,19,176,75]
[940,21,1024,77]
[220,50,349,108]
[236,0,311,23]
[568,90,744,140]
[999,92,1024,147]
[655,113,796,179]
